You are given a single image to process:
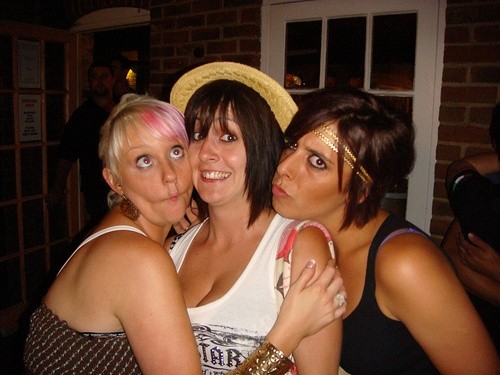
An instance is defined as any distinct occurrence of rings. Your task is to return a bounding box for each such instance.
[334,294,345,306]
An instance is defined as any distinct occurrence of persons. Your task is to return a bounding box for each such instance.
[439,101,500,360]
[46,55,130,219]
[163,61,342,375]
[21,93,347,375]
[172,83,500,375]
